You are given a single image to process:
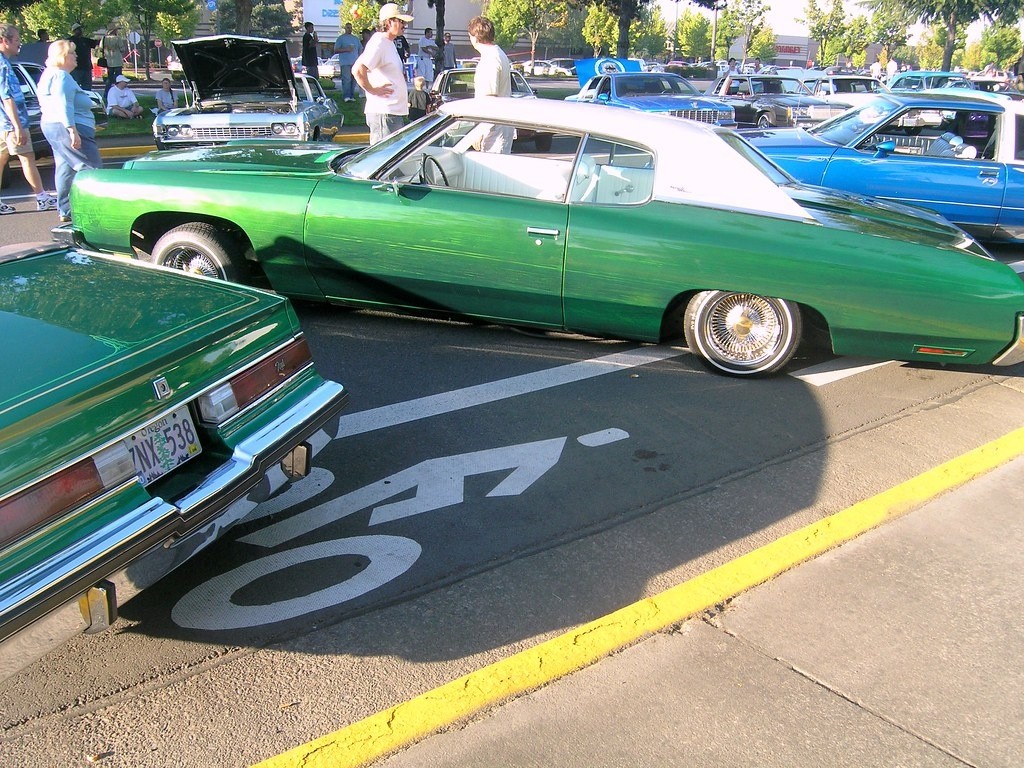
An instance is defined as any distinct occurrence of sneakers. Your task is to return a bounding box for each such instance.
[36,194,57,211]
[0,199,16,214]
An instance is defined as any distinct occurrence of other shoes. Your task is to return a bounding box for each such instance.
[134,115,143,119]
[59,211,71,222]
[350,98,357,102]
[344,97,350,102]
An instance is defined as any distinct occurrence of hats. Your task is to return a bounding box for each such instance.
[359,29,370,34]
[116,75,131,83]
[105,23,122,35]
[379,3,415,22]
[71,23,83,31]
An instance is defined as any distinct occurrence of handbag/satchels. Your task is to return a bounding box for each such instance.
[97,58,108,67]
[737,68,743,83]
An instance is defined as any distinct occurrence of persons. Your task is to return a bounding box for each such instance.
[302,22,319,80]
[984,61,994,75]
[36,40,103,222]
[408,76,431,124]
[106,75,143,119]
[417,28,439,90]
[352,2,415,144]
[155,78,179,113]
[886,57,898,82]
[393,35,411,64]
[35,29,53,66]
[359,28,377,97]
[66,23,101,91]
[99,23,125,100]
[870,59,882,90]
[468,16,514,153]
[334,23,364,102]
[900,61,907,72]
[444,33,458,70]
[722,58,743,95]
[0,23,56,214]
[750,57,766,93]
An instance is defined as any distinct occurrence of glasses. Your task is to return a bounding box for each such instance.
[393,19,405,24]
[446,35,451,37]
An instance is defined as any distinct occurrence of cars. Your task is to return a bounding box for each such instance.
[407,55,482,83]
[66,96,1024,381]
[427,68,540,142]
[165,57,185,73]
[795,76,925,135]
[705,73,856,132]
[971,70,1018,92]
[629,58,855,73]
[0,242,353,684]
[9,61,108,141]
[551,57,577,75]
[563,57,738,131]
[292,54,341,81]
[720,95,1024,244]
[887,72,991,125]
[522,60,573,77]
[151,34,344,153]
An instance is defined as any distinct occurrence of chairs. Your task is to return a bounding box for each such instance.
[647,82,661,93]
[739,82,749,92]
[450,83,468,93]
[924,132,977,159]
[539,155,596,200]
